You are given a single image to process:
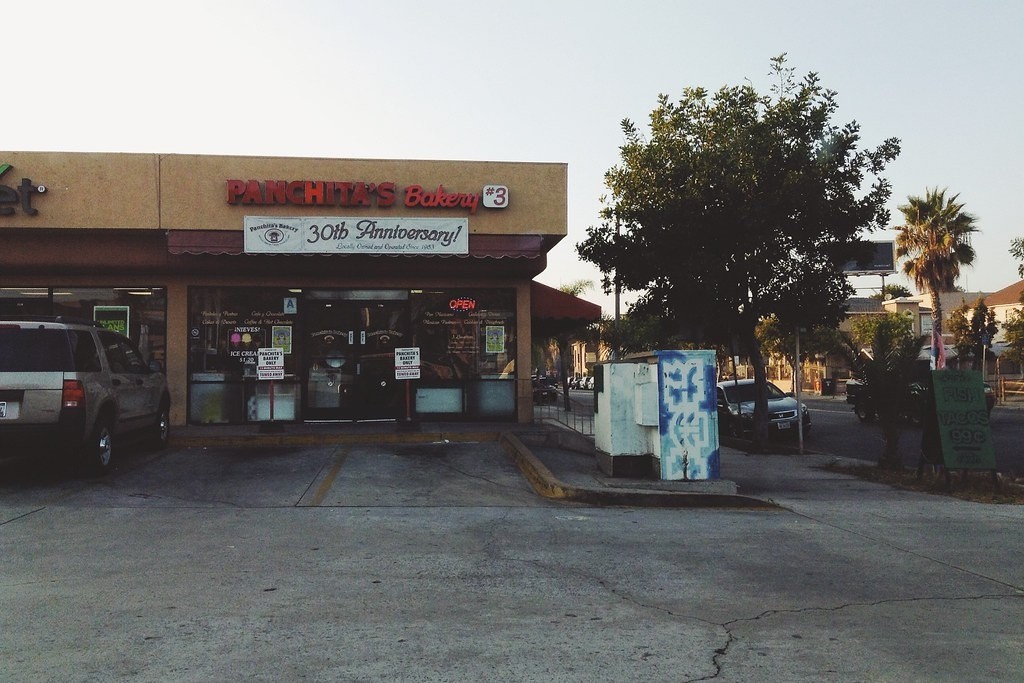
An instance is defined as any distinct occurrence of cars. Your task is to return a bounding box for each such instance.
[717,378,812,444]
[846,346,997,425]
[567,376,594,390]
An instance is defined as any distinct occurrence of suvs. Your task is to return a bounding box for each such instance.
[0,315,172,476]
